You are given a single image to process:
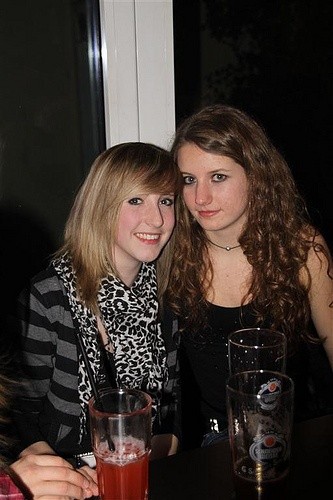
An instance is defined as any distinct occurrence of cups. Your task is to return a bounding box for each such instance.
[88,387,153,500]
[225,370,295,484]
[227,327,287,454]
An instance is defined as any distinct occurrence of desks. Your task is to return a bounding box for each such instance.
[84,413,333,500]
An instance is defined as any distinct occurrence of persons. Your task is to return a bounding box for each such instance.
[166,106,332,499]
[0,142,212,500]
